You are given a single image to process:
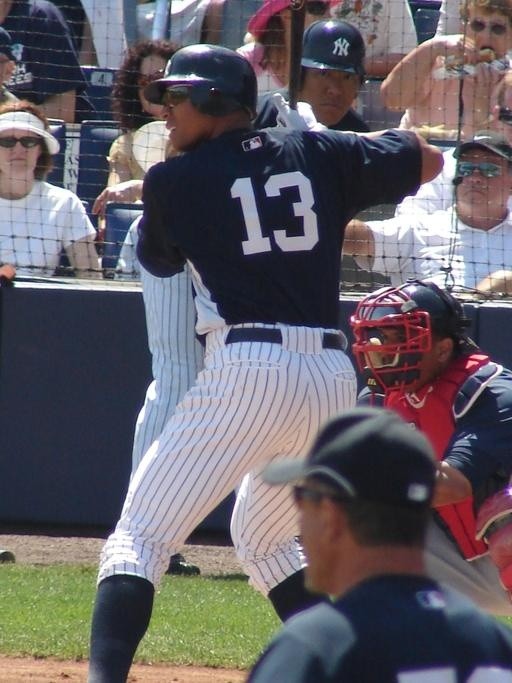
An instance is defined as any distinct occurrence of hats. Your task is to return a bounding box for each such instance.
[453,131,512,161]
[248,0,339,36]
[264,407,435,510]
[0,111,60,155]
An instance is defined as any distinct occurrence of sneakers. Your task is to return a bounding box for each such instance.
[165,553,200,574]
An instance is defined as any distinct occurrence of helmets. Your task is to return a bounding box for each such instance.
[301,19,364,74]
[357,277,462,391]
[143,44,257,120]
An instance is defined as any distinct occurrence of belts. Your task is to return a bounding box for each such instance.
[225,328,346,350]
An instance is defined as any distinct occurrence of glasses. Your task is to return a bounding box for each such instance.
[161,85,189,108]
[457,161,501,178]
[470,19,506,35]
[0,136,41,147]
[305,1,327,15]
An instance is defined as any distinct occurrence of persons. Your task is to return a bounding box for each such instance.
[338,280,510,618]
[1,1,511,306]
[86,42,444,682]
[327,129,512,300]
[244,407,510,681]
[128,18,367,577]
